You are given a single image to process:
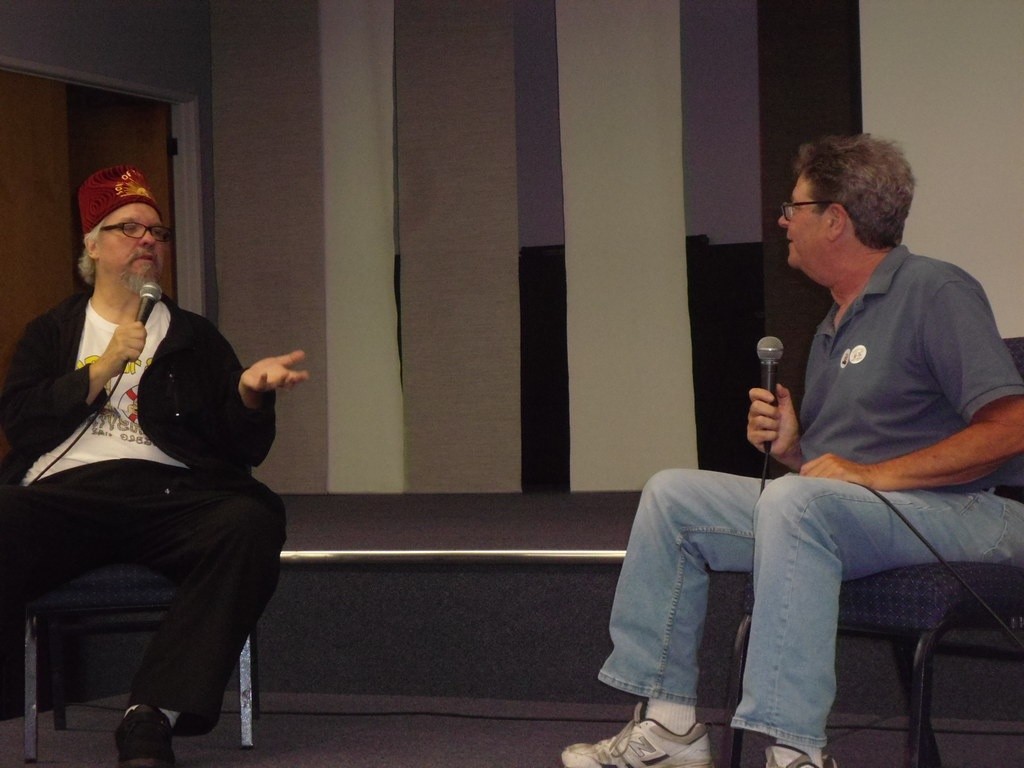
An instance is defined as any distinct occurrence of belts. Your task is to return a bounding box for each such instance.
[984,485,1024,504]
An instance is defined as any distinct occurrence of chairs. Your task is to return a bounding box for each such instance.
[0,463,261,764]
[719,337,1024,768]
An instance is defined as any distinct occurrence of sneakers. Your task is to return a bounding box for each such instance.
[560,702,715,768]
[765,743,837,768]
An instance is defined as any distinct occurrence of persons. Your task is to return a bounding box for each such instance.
[563,134,1024,768]
[0,164,308,768]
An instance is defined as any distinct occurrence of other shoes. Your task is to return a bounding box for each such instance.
[114,704,175,768]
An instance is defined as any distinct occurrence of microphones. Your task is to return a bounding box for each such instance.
[135,282,163,328]
[756,337,783,452]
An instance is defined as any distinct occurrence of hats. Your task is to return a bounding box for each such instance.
[77,165,162,234]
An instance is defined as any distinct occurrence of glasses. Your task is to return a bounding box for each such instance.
[100,222,173,242]
[781,200,846,221]
